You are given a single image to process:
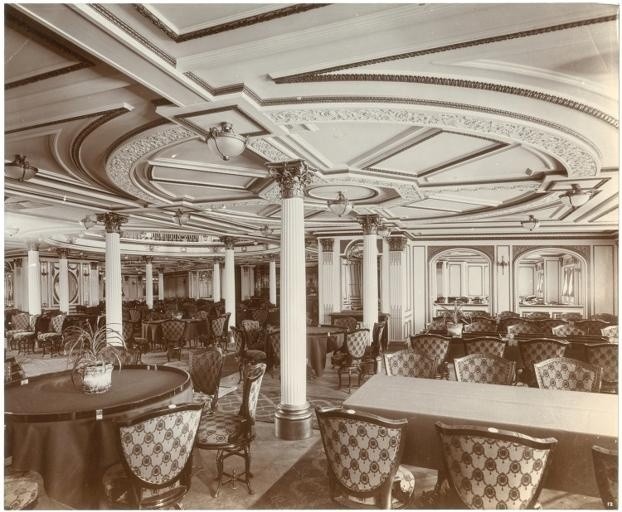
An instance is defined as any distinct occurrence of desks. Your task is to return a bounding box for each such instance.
[342,373,618,508]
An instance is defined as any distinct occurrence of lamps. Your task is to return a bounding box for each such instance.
[376,224,392,240]
[326,188,356,218]
[4,154,40,182]
[173,207,191,226]
[260,224,273,237]
[79,215,98,230]
[557,183,591,207]
[520,214,542,231]
[205,122,249,164]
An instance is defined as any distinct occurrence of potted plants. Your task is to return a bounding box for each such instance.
[61,315,128,394]
[439,298,471,338]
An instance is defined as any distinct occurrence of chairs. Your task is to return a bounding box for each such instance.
[382,309,618,395]
[4,299,231,360]
[591,444,618,508]
[229,298,388,392]
[101,402,205,508]
[314,405,415,507]
[3,471,42,509]
[3,359,26,386]
[188,347,221,412]
[186,363,267,498]
[433,421,558,507]
